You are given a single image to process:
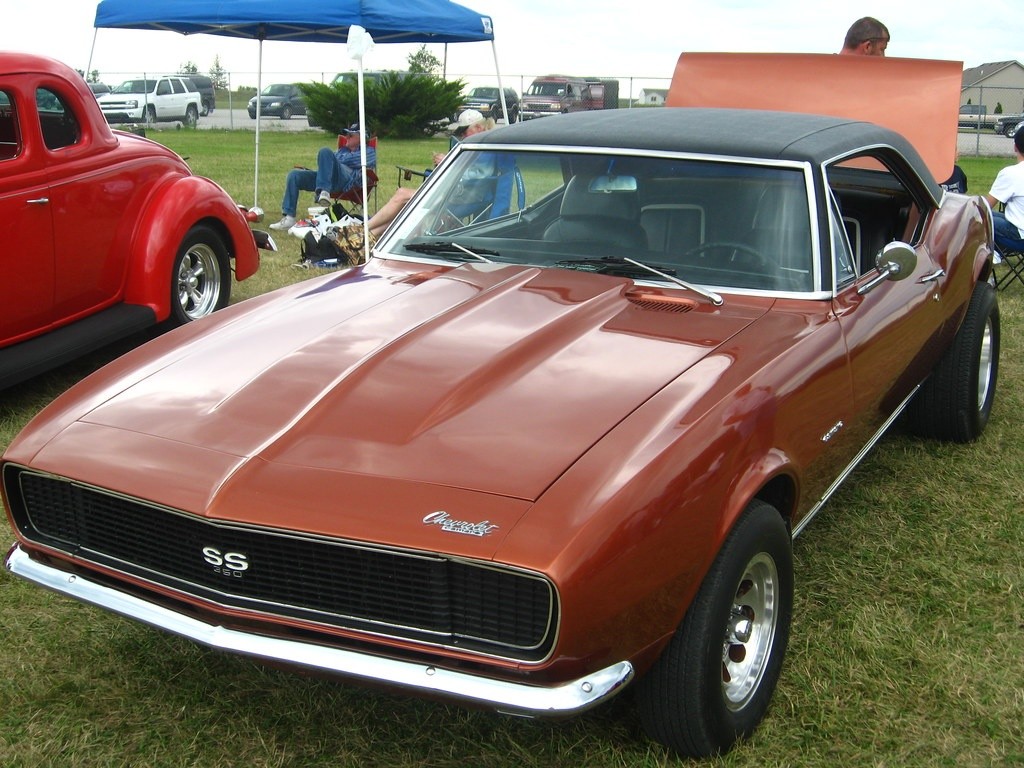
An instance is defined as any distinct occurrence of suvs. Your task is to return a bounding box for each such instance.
[163,74,216,117]
[95,76,203,129]
[449,87,519,125]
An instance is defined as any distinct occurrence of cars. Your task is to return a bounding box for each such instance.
[0,52,1001,759]
[0,50,263,397]
[994,112,1024,138]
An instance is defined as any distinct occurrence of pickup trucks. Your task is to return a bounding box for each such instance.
[957,104,1021,128]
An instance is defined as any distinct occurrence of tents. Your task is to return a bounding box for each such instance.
[85,0,509,264]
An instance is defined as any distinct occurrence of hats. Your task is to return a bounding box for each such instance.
[447,109,483,130]
[342,122,369,138]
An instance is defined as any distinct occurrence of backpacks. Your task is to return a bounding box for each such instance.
[299,230,348,267]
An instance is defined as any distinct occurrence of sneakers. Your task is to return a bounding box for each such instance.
[269,216,295,231]
[318,192,331,207]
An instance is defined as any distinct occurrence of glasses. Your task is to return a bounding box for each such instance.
[458,126,469,134]
[345,134,359,137]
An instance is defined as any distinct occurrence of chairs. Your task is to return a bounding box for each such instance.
[293,163,379,215]
[988,201,1024,292]
[424,150,516,229]
[731,185,815,281]
[541,172,648,249]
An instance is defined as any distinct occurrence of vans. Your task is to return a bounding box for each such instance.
[521,76,603,121]
[248,84,306,120]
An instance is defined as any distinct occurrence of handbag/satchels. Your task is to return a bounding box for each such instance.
[335,224,377,264]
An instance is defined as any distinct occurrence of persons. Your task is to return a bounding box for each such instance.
[939,152,968,193]
[832,17,889,56]
[363,109,500,235]
[988,125,1024,252]
[269,122,375,231]
